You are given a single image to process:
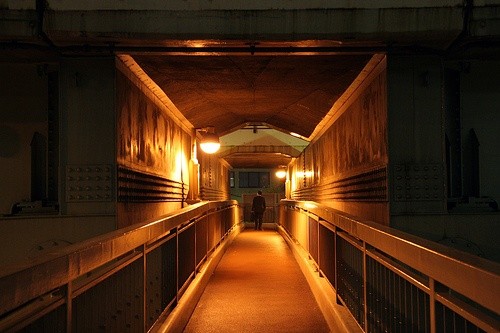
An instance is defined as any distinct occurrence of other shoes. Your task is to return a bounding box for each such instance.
[254,226,262,230]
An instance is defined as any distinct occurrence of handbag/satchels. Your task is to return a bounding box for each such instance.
[251,212,254,222]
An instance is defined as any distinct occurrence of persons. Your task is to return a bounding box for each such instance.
[251,190,266,231]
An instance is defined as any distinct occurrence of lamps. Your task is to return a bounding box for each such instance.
[191,124,221,161]
[273,165,289,178]
